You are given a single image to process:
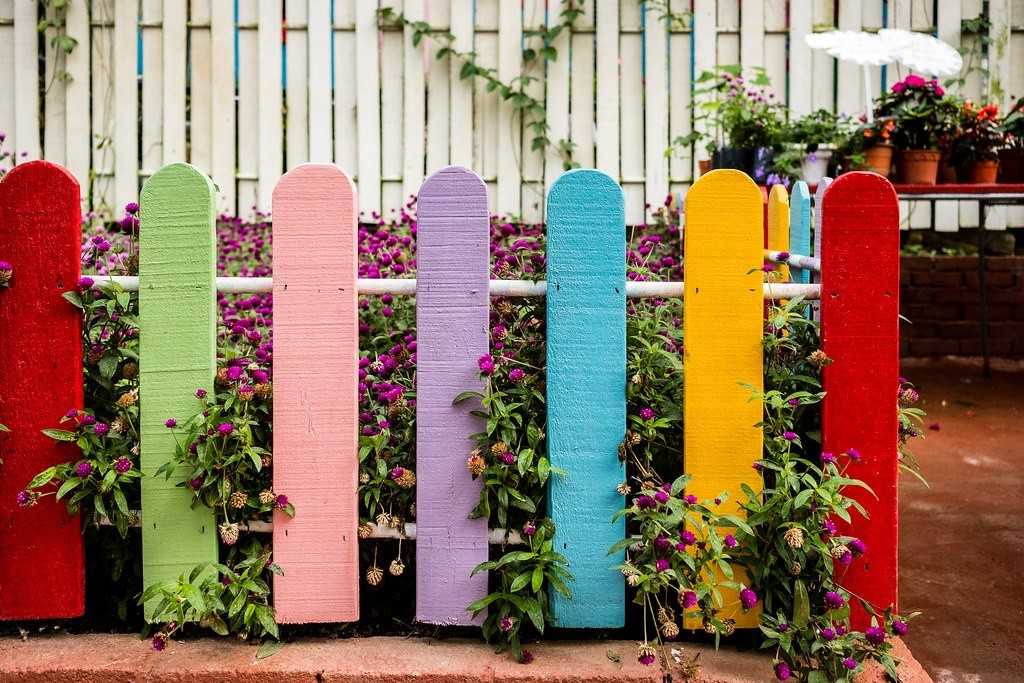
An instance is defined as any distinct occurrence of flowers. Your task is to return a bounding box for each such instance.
[659,56,1022,186]
